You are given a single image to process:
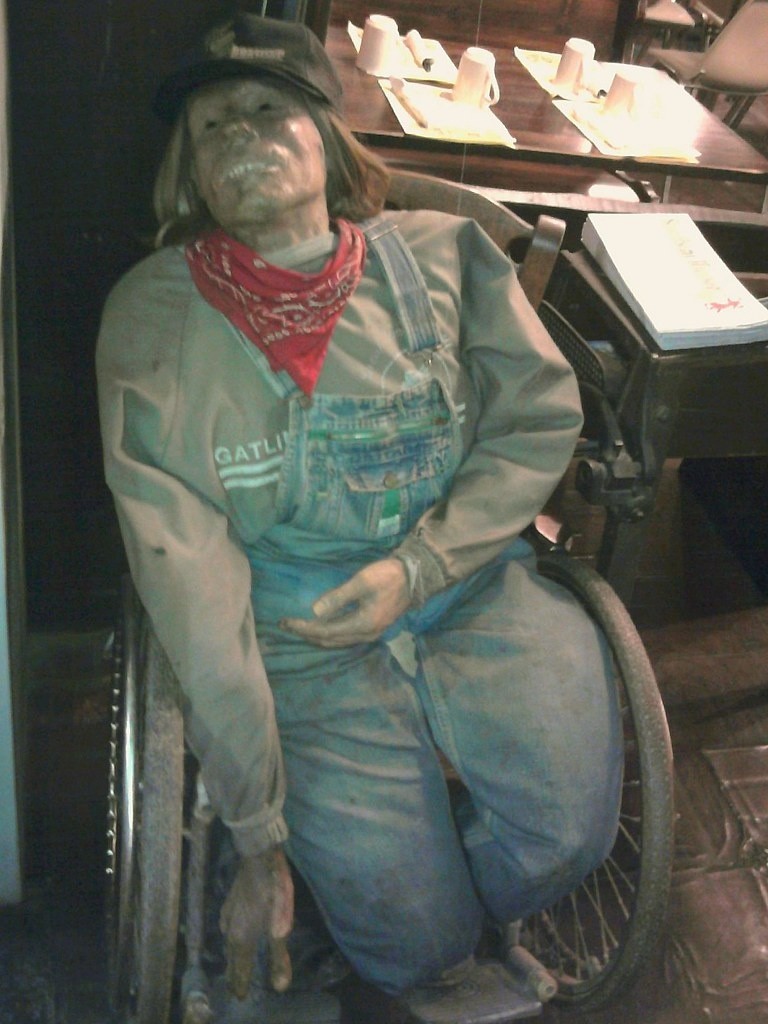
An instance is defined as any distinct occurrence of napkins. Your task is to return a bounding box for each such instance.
[347,20,702,164]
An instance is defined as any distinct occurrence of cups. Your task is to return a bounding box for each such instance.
[599,70,641,121]
[354,12,402,77]
[552,36,597,97]
[451,45,502,110]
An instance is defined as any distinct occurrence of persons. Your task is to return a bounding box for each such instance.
[95,12,624,1000]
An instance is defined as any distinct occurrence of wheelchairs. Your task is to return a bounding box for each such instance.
[77,161,684,1024]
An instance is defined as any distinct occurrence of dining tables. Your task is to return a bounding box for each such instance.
[321,26,768,203]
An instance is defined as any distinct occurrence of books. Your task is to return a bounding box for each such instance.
[578,213,768,350]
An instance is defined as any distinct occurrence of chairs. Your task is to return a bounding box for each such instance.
[627,0,768,129]
